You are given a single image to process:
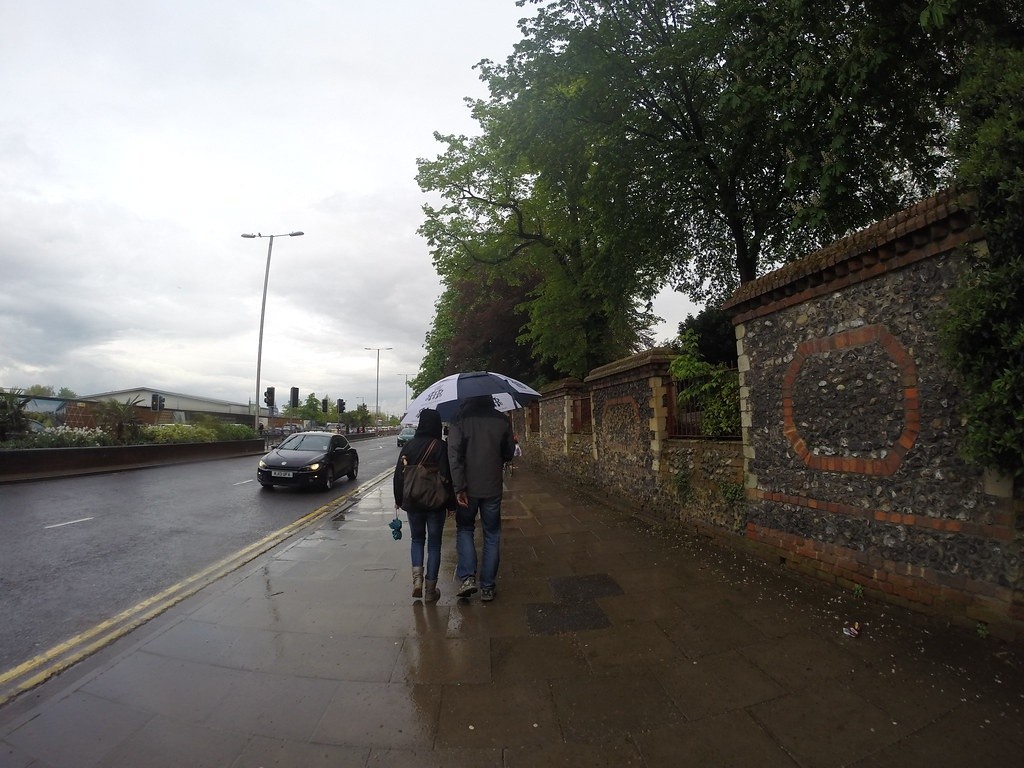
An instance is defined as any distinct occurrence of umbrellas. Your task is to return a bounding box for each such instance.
[400,369,542,424]
[389,507,402,540]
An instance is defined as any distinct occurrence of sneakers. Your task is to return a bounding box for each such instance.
[456,575,478,598]
[481,582,497,601]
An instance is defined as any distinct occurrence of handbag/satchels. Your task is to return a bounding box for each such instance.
[401,438,451,513]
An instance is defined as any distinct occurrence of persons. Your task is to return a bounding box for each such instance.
[258,423,264,434]
[447,395,515,601]
[393,410,455,603]
[361,427,365,433]
[357,427,360,433]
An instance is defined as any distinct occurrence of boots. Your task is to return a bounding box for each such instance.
[411,566,424,598]
[425,574,441,605]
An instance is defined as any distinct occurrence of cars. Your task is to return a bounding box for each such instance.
[0,415,48,440]
[257,431,359,492]
[397,427,416,447]
[260,422,417,437]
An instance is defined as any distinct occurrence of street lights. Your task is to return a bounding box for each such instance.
[241,231,305,432]
[397,373,417,411]
[364,347,393,434]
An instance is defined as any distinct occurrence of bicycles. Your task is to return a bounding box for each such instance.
[503,459,514,478]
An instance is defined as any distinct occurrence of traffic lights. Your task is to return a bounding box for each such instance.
[322,399,328,412]
[152,395,160,411]
[337,398,346,414]
